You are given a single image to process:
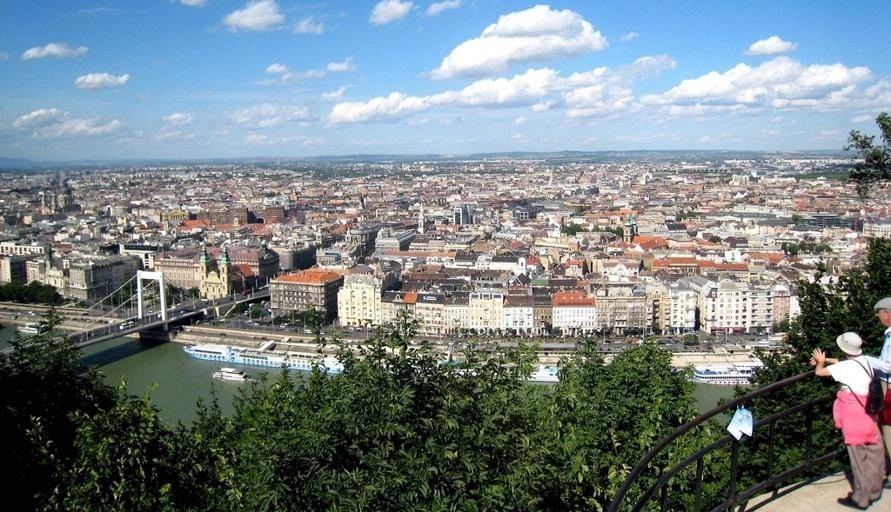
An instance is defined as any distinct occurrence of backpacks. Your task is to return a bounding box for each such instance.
[848,355,885,414]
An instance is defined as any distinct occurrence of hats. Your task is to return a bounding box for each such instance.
[873,298,891,311]
[837,332,863,356]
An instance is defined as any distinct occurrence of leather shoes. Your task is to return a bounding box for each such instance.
[837,479,891,509]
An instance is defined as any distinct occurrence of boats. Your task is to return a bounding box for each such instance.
[16,320,50,337]
[683,362,765,384]
[210,368,250,382]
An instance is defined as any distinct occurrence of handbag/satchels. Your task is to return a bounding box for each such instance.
[880,389,891,425]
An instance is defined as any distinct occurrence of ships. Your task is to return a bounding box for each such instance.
[182,345,343,375]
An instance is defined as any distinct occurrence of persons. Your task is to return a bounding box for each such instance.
[863,297,891,489]
[809,330,888,509]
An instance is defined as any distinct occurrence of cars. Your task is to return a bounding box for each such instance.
[3,305,119,323]
[83,331,96,337]
[119,284,276,333]
